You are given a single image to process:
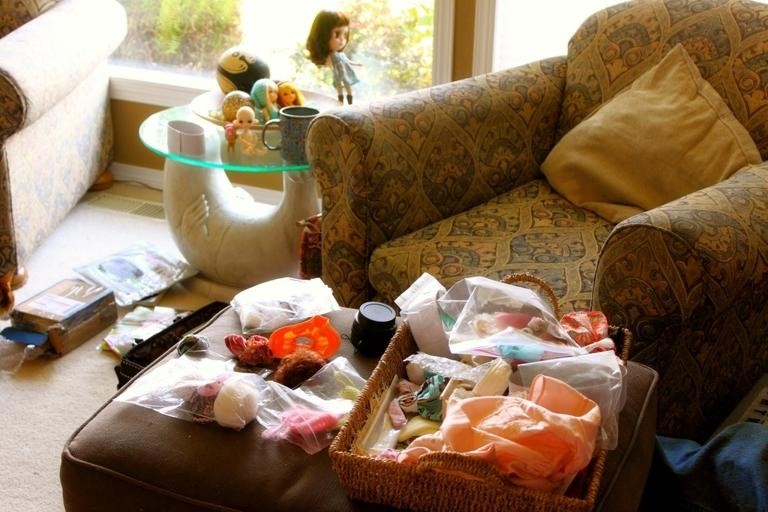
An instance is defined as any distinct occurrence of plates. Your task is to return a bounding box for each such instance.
[190,87,345,131]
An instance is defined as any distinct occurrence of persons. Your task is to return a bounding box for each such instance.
[304,10,363,104]
[214,42,304,157]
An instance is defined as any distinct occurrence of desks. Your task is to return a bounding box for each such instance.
[136,89,364,306]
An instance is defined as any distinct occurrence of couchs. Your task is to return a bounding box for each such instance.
[303,0,767,439]
[58,287,661,512]
[0,0,133,320]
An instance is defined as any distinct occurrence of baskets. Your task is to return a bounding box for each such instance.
[330,272,633,511]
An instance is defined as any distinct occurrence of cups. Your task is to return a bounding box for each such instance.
[259,104,322,163]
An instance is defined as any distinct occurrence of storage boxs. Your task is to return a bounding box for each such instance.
[6,274,119,356]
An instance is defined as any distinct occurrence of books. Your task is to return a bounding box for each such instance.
[75,242,199,305]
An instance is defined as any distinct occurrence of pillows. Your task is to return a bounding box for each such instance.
[541,39,764,228]
